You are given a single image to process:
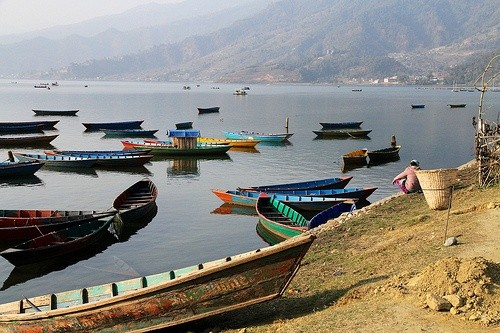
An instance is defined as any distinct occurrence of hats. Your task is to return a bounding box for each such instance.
[410,159,419,166]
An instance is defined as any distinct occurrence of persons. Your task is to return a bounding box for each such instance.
[391,136,396,147]
[392,160,422,194]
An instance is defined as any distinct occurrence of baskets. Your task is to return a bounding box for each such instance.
[414,169,458,210]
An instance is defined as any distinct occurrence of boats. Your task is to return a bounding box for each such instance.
[32,110,79,114]
[367,145,402,161]
[312,129,372,136]
[411,104,425,107]
[320,121,363,127]
[0,234,317,333]
[341,148,368,166]
[197,106,220,111]
[113,178,158,224]
[450,104,467,107]
[0,210,118,266]
[208,176,378,240]
[0,117,295,175]
